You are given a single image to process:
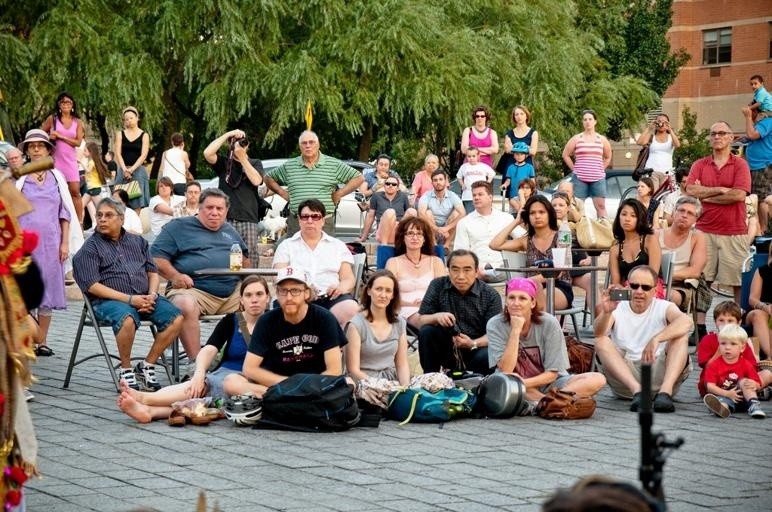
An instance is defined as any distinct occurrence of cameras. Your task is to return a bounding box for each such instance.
[449,322,463,337]
[653,121,663,130]
[230,135,250,149]
[608,289,630,302]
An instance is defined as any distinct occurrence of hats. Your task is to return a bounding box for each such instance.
[16,129,56,157]
[274,265,305,286]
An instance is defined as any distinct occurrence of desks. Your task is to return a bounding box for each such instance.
[195,268,279,280]
[495,266,608,317]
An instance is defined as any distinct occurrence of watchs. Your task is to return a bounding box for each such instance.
[471,341,477,351]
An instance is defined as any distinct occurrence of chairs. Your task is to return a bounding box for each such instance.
[376,245,445,351]
[740,253,768,315]
[63,273,174,393]
[501,249,584,342]
[590,251,676,372]
[342,252,366,373]
[172,314,227,382]
[355,190,417,239]
[685,278,699,352]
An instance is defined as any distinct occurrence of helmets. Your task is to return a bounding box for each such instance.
[511,142,529,153]
[221,395,261,426]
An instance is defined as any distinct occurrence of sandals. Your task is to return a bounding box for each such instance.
[35,346,54,356]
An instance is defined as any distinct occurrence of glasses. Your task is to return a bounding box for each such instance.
[60,101,73,105]
[404,231,425,235]
[385,182,398,186]
[709,130,732,137]
[627,283,655,292]
[476,114,486,118]
[299,214,325,221]
[278,288,309,295]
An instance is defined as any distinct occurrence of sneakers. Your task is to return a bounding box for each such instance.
[563,397,599,418]
[704,393,728,419]
[747,399,767,419]
[357,202,369,211]
[134,360,160,392]
[168,407,211,427]
[118,366,141,393]
[24,390,35,403]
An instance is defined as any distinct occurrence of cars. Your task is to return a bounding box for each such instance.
[448,175,553,215]
[551,169,647,224]
[175,159,409,236]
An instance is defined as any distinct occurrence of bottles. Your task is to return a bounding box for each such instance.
[230,241,242,272]
[555,217,573,269]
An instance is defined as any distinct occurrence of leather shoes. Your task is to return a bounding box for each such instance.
[654,392,676,411]
[630,390,646,411]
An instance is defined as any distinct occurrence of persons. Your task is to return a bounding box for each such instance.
[117,275,270,424]
[224,268,347,397]
[700,324,767,417]
[598,265,694,412]
[594,76,772,344]
[415,249,501,374]
[193,126,417,310]
[405,102,610,326]
[2,97,193,511]
[345,269,411,406]
[698,300,769,400]
[486,276,606,401]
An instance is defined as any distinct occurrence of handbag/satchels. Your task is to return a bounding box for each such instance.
[114,181,142,201]
[258,196,272,222]
[565,335,595,373]
[576,218,617,248]
[632,145,651,181]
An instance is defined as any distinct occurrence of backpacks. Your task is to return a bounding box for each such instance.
[253,373,361,433]
[389,385,475,424]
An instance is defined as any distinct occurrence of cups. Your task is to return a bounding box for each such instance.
[551,247,566,268]
